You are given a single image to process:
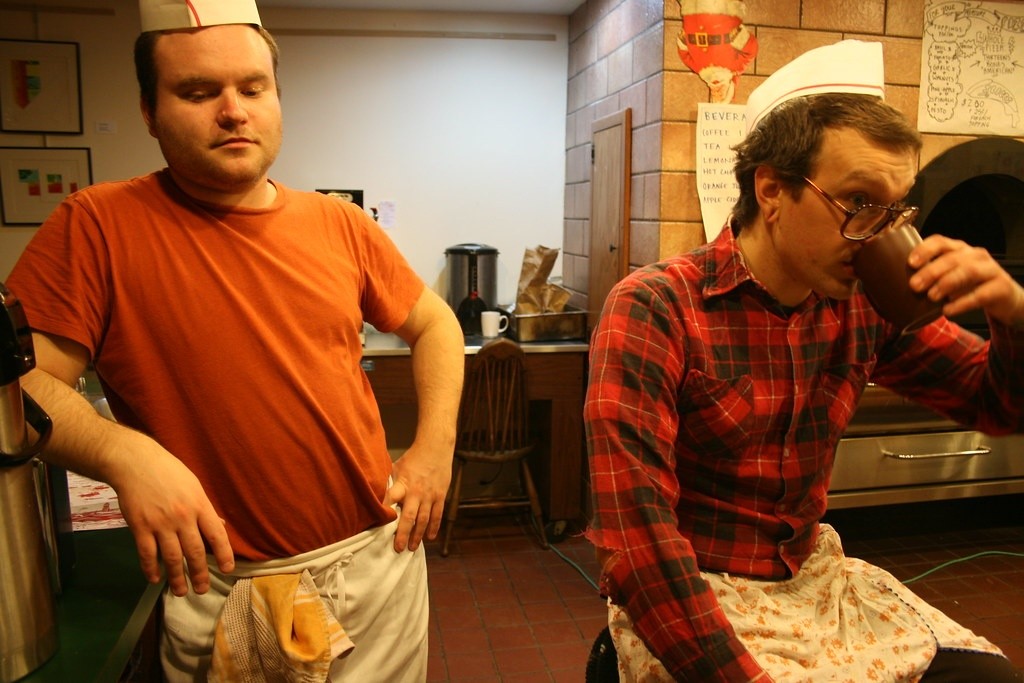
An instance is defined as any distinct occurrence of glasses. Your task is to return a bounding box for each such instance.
[777,171,920,241]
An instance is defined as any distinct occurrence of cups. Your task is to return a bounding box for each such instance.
[852,223,951,333]
[481,310,509,338]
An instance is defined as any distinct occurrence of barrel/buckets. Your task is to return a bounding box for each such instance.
[0,285,68,683]
[445,244,500,336]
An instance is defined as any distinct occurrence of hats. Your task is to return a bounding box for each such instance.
[139,0,262,34]
[739,40,884,139]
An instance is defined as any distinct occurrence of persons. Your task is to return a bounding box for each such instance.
[4,0,465,683]
[580,39,1024,683]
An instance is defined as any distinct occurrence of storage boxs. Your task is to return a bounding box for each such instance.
[497,304,588,343]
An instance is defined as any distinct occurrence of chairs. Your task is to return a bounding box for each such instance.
[441,338,548,557]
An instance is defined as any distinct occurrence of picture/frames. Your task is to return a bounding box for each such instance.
[0,38,84,135]
[0,146,93,225]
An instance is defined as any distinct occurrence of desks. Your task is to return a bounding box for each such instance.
[360,332,594,544]
